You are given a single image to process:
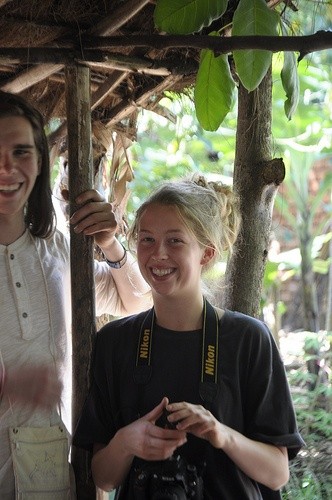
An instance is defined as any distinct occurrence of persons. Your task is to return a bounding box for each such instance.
[0,88,155,500]
[77,170,307,500]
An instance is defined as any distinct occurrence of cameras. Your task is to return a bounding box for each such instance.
[130,448,203,500]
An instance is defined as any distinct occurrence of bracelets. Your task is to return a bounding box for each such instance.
[105,245,127,269]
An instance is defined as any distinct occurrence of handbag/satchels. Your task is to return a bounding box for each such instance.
[9,424,75,499]
